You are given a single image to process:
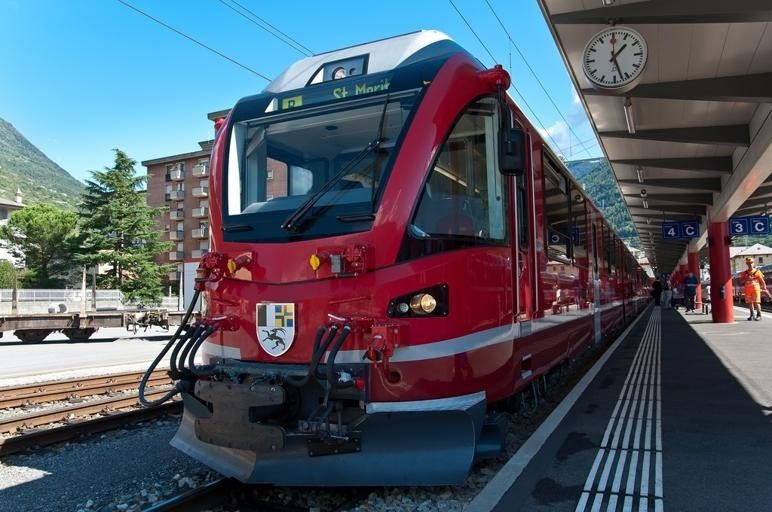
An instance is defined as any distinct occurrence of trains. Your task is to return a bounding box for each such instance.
[139,29,655,488]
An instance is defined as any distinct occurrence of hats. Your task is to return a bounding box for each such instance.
[746,258,754,263]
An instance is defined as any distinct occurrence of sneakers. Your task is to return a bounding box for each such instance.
[748,316,761,321]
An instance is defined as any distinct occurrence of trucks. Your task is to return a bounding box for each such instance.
[1,310,126,343]
[182,261,202,331]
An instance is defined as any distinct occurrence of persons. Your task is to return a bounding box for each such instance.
[552,276,620,312]
[651,271,712,315]
[738,257,766,321]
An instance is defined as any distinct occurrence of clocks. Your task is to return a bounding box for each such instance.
[581,26,649,95]
